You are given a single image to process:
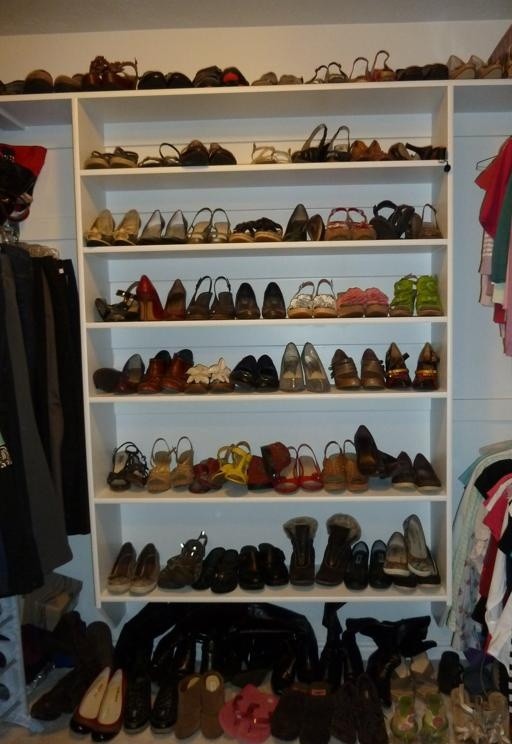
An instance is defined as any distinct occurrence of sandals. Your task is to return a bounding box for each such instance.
[306,50,503,84]
[287,274,443,318]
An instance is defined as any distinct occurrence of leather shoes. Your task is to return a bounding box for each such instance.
[95,274,286,321]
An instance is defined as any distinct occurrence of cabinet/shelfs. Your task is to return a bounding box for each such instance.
[74,81,454,610]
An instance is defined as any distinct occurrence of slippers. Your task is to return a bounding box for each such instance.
[483,691,510,744]
[438,651,464,697]
[450,688,487,744]
